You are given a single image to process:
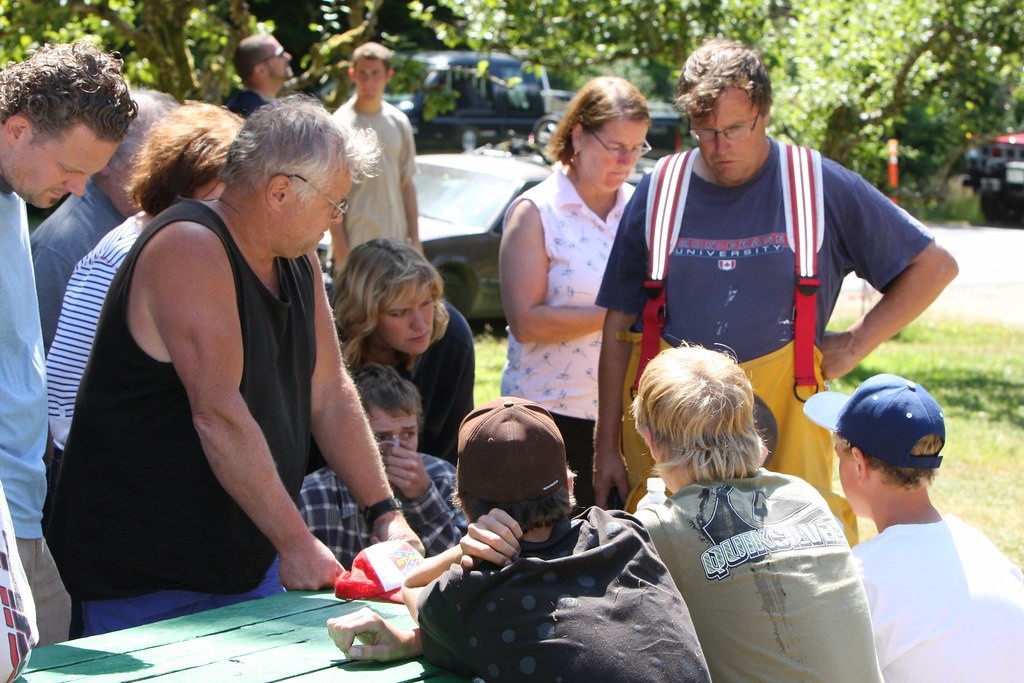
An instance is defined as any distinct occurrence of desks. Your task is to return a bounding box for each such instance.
[9,586,480,683]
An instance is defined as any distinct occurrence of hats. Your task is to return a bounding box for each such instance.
[803,372,945,469]
[457,396,567,504]
[335,539,430,604]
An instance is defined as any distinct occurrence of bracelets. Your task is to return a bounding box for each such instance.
[364,498,402,526]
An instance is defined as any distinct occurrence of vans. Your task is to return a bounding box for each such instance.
[383,51,550,153]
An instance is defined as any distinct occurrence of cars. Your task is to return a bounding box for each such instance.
[645,99,683,159]
[318,148,662,326]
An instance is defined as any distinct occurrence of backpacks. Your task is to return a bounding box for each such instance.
[0,479,41,683]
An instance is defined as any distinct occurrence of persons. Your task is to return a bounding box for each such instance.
[46,96,425,642]
[401,397,710,682]
[0,42,138,648]
[593,41,958,549]
[332,238,475,467]
[330,42,424,274]
[498,77,653,521]
[300,362,469,572]
[31,87,247,538]
[629,346,883,683]
[803,374,1022,682]
[224,35,295,120]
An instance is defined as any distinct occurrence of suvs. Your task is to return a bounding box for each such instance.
[959,116,1024,225]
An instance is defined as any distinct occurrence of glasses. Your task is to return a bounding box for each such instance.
[687,104,762,143]
[283,175,351,220]
[262,45,284,64]
[583,124,653,158]
